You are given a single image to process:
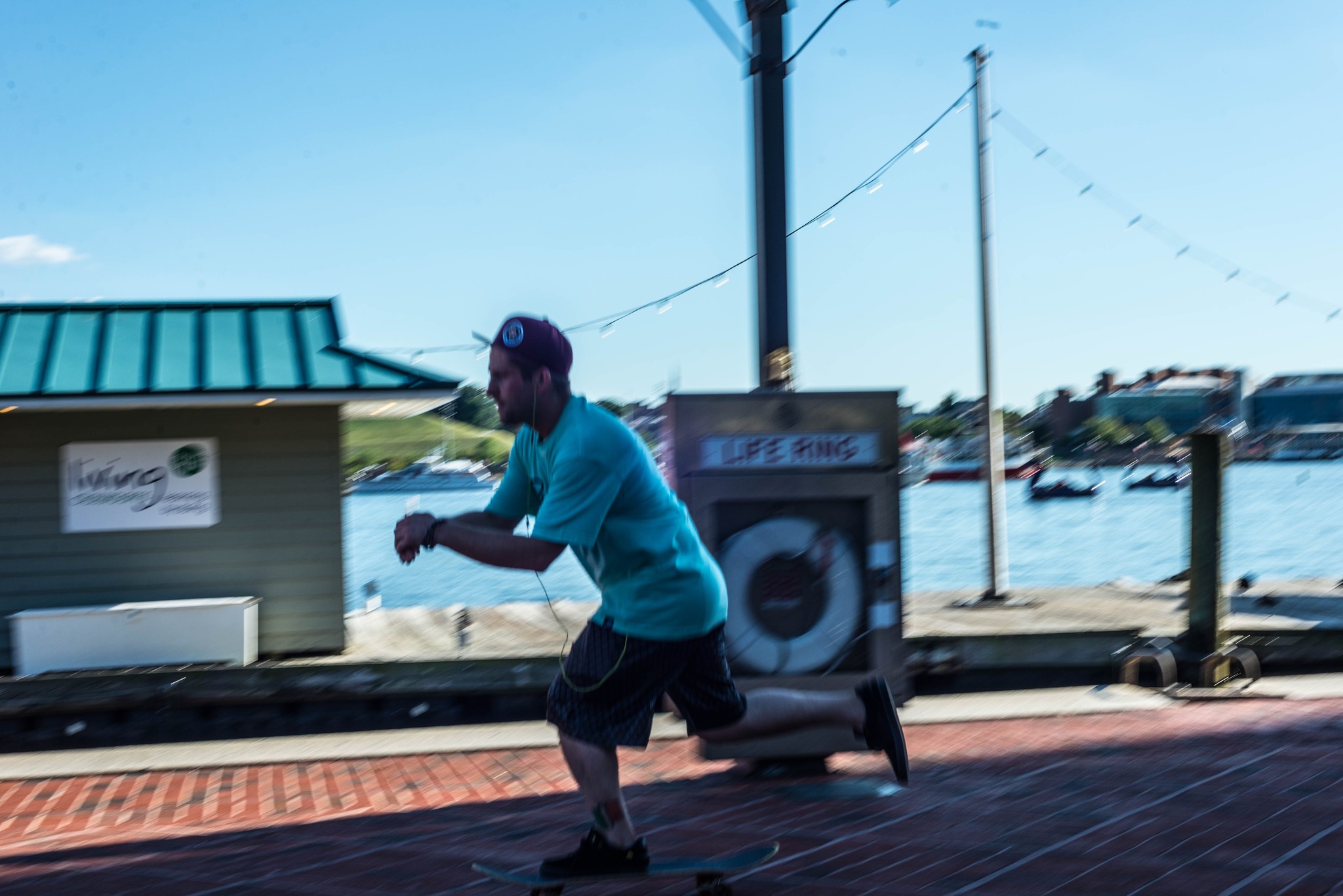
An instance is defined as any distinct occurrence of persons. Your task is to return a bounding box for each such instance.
[395,315,908,880]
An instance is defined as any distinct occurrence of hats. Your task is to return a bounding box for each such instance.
[472,316,574,375]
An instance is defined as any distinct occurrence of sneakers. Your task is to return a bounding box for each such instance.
[853,672,911,787]
[540,829,654,877]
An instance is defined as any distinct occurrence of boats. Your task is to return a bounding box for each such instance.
[338,440,510,499]
[898,426,1191,501]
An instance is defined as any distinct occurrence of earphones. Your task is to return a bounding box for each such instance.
[536,375,543,388]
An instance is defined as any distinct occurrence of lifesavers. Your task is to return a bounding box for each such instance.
[723,518,862,675]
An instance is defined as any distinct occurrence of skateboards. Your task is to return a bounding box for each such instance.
[471,841,780,896]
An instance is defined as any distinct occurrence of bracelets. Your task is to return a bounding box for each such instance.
[422,518,447,550]
